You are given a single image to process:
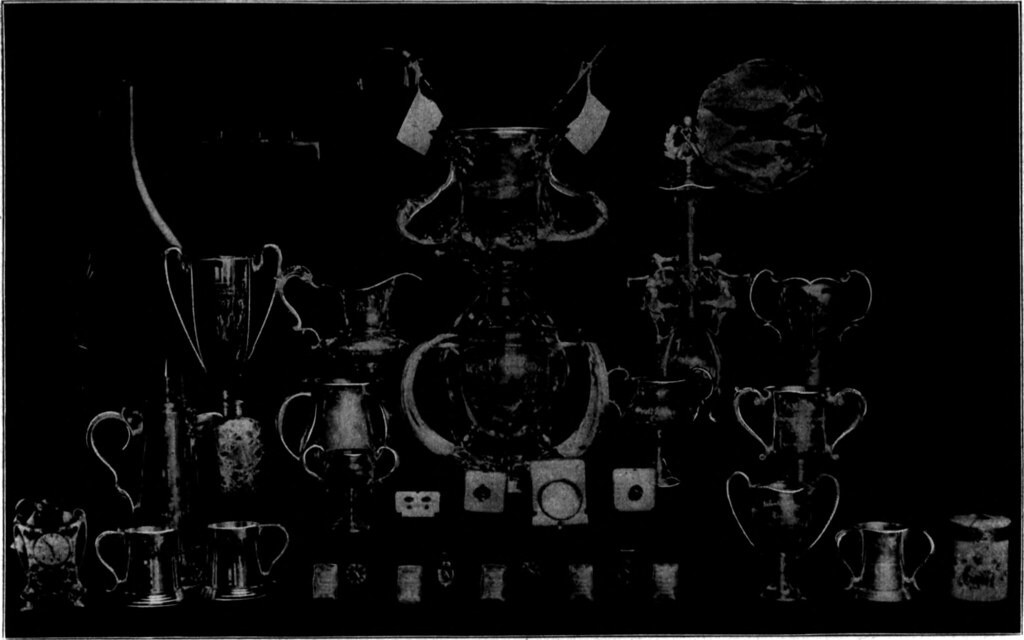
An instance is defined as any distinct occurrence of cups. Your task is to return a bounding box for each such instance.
[208,521,290,601]
[94,526,185,607]
